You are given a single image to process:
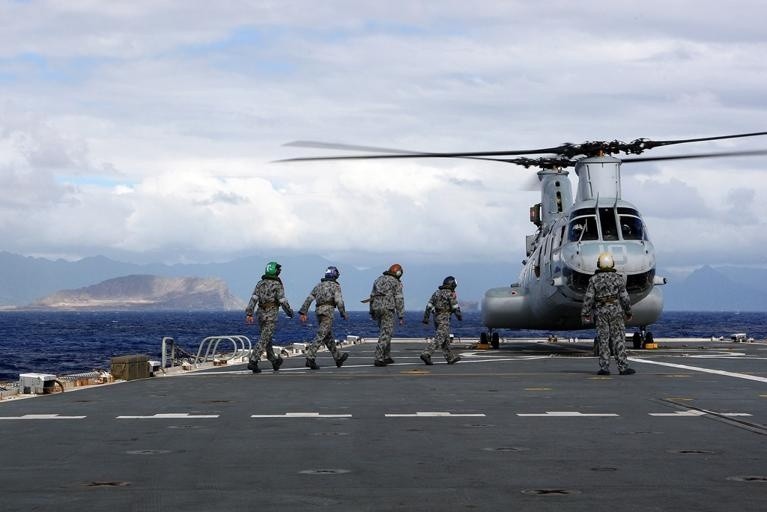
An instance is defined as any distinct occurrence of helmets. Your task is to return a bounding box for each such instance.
[325,266,340,279]
[597,254,614,269]
[443,276,457,289]
[265,262,281,276]
[390,264,403,278]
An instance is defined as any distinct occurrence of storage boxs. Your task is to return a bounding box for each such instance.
[111,355,150,380]
[19,372,57,393]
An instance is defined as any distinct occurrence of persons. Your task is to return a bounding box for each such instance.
[298,266,349,370]
[581,253,635,375]
[570,224,586,241]
[620,224,631,240]
[420,276,463,364]
[369,264,405,367]
[245,262,294,373]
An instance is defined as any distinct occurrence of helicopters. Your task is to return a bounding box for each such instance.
[270,131,766,348]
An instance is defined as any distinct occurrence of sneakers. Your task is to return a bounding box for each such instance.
[248,362,261,372]
[448,357,460,364]
[336,353,348,367]
[620,368,635,374]
[306,360,320,369]
[420,354,433,365]
[598,369,609,375]
[273,357,283,370]
[375,358,394,366]
